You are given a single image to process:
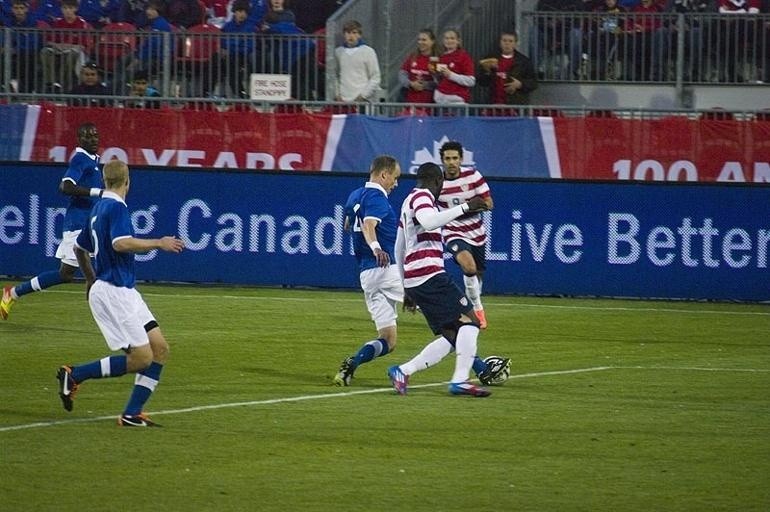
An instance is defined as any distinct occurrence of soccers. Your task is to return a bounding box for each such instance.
[483,357,510,384]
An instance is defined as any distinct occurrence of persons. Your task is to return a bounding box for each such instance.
[475,32,540,116]
[538,1,763,83]
[429,29,477,114]
[384,164,494,398]
[331,21,379,114]
[0,0,329,117]
[57,160,185,428]
[331,153,511,386]
[437,142,496,330]
[395,27,437,115]
[1,123,104,318]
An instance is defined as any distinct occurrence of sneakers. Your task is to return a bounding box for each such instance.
[474,309,487,329]
[447,378,491,397]
[334,357,354,387]
[119,412,162,427]
[388,365,409,395]
[476,358,512,385]
[56,365,80,412]
[0,285,18,320]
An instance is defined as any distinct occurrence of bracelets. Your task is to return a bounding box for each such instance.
[460,201,470,213]
[88,187,101,197]
[368,238,383,253]
